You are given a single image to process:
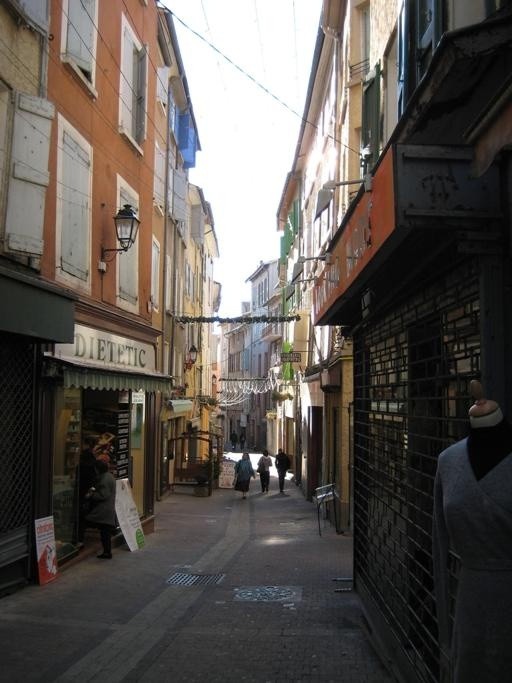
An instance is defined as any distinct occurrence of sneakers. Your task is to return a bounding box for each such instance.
[242,496,246,499]
[266,489,268,492]
[96,551,113,560]
[75,541,85,548]
[262,489,265,492]
[280,490,283,493]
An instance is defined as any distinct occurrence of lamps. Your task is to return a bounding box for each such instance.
[313,172,375,222]
[284,275,319,301]
[183,344,198,366]
[102,203,143,259]
[292,250,332,283]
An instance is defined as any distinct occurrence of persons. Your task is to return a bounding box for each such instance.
[427,380,512,683]
[234,452,256,499]
[257,448,273,493]
[274,448,287,494]
[230,429,238,450]
[239,431,246,451]
[84,460,117,560]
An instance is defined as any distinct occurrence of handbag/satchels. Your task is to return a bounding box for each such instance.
[257,458,265,474]
